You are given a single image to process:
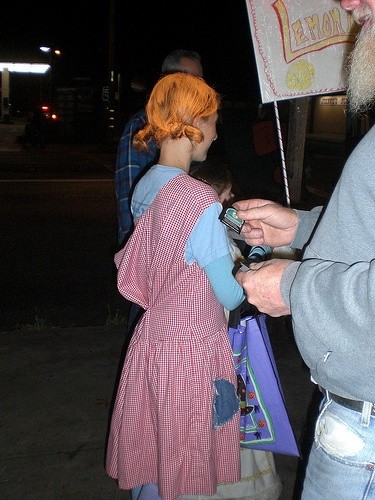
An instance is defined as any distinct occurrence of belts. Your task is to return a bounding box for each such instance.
[323,388,374,415]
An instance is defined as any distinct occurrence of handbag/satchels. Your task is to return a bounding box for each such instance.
[229,315,302,459]
